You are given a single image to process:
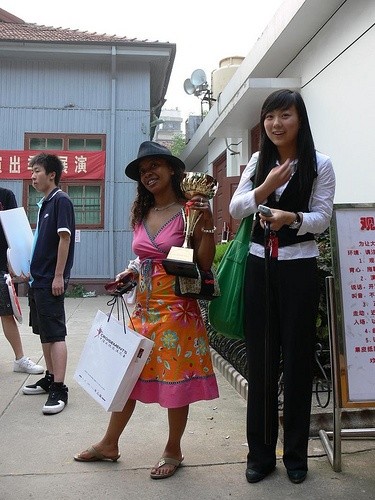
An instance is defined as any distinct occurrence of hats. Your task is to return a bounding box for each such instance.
[124,141,185,182]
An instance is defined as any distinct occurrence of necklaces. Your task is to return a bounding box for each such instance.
[153,198,179,210]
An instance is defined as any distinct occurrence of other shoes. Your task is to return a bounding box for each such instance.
[246,468,264,483]
[288,472,306,484]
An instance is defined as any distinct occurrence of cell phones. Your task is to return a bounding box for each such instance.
[111,280,137,296]
[257,205,273,217]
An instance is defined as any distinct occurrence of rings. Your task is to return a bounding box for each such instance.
[201,198,203,202]
[200,203,203,207]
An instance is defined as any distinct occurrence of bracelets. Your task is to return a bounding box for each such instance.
[201,226,217,233]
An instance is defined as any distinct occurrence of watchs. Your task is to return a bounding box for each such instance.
[289,212,301,229]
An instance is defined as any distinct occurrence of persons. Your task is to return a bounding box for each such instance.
[72,141,220,480]
[228,89,335,484]
[0,188,43,373]
[21,154,75,414]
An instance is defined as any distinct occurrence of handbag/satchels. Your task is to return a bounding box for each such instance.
[207,212,254,341]
[174,261,222,301]
[74,292,155,413]
[4,274,23,325]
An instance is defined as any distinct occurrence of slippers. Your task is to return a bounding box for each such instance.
[73,445,121,462]
[150,454,185,479]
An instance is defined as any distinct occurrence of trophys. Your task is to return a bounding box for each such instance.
[162,172,219,279]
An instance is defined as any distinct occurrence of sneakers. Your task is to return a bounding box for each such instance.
[14,355,45,374]
[22,369,54,394]
[42,382,68,413]
